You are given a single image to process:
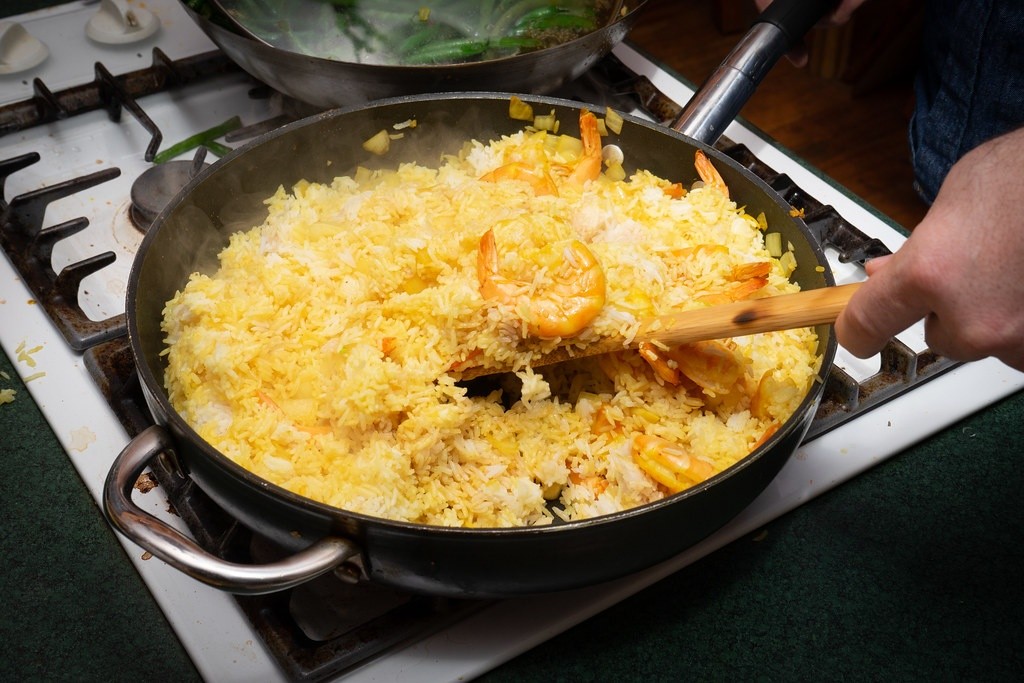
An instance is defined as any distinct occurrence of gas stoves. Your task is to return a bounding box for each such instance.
[0,0,1024,683]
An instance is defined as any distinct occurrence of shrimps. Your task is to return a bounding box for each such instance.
[630,434,714,494]
[477,109,601,206]
[747,369,798,454]
[477,227,606,339]
[639,338,740,394]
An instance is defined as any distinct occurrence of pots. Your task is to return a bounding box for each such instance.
[174,0,654,109]
[99,0,840,598]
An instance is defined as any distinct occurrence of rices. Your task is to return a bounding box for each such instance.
[157,130,822,529]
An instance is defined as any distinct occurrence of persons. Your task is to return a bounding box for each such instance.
[755,0,1024,376]
